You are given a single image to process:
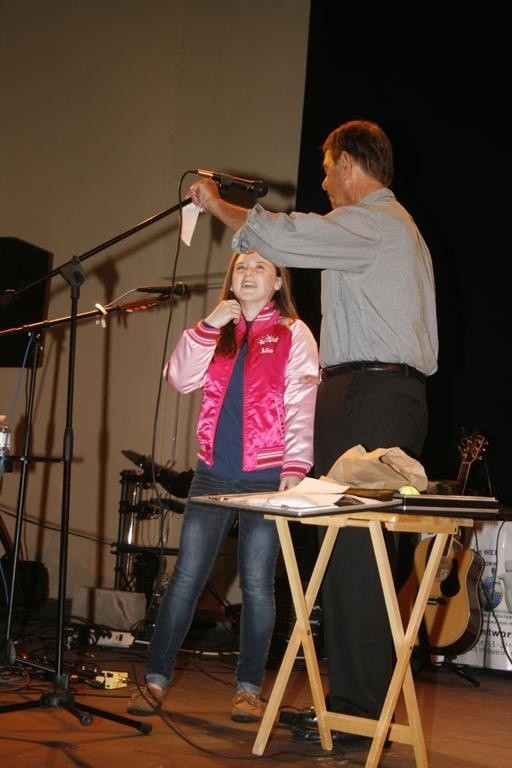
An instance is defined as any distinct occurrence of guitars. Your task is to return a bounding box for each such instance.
[397,435,485,656]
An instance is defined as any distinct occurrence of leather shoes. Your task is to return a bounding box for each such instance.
[279,704,329,722]
[294,726,393,748]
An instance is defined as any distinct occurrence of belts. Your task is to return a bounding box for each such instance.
[319,358,428,385]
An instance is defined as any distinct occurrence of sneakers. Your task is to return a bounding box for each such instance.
[231,686,262,722]
[124,678,173,718]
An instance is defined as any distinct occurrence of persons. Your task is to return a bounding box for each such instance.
[130,240,318,724]
[189,120,441,743]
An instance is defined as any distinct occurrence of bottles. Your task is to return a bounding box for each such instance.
[0,414,11,457]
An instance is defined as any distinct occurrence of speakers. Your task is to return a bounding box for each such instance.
[0,237,50,367]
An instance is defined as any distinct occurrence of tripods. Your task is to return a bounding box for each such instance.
[0,197,193,734]
[0,332,59,677]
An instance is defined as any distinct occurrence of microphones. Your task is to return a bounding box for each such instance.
[196,168,268,197]
[138,281,188,296]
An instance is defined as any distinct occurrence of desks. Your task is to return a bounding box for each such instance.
[246,507,478,768]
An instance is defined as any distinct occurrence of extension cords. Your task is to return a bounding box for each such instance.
[74,627,135,648]
